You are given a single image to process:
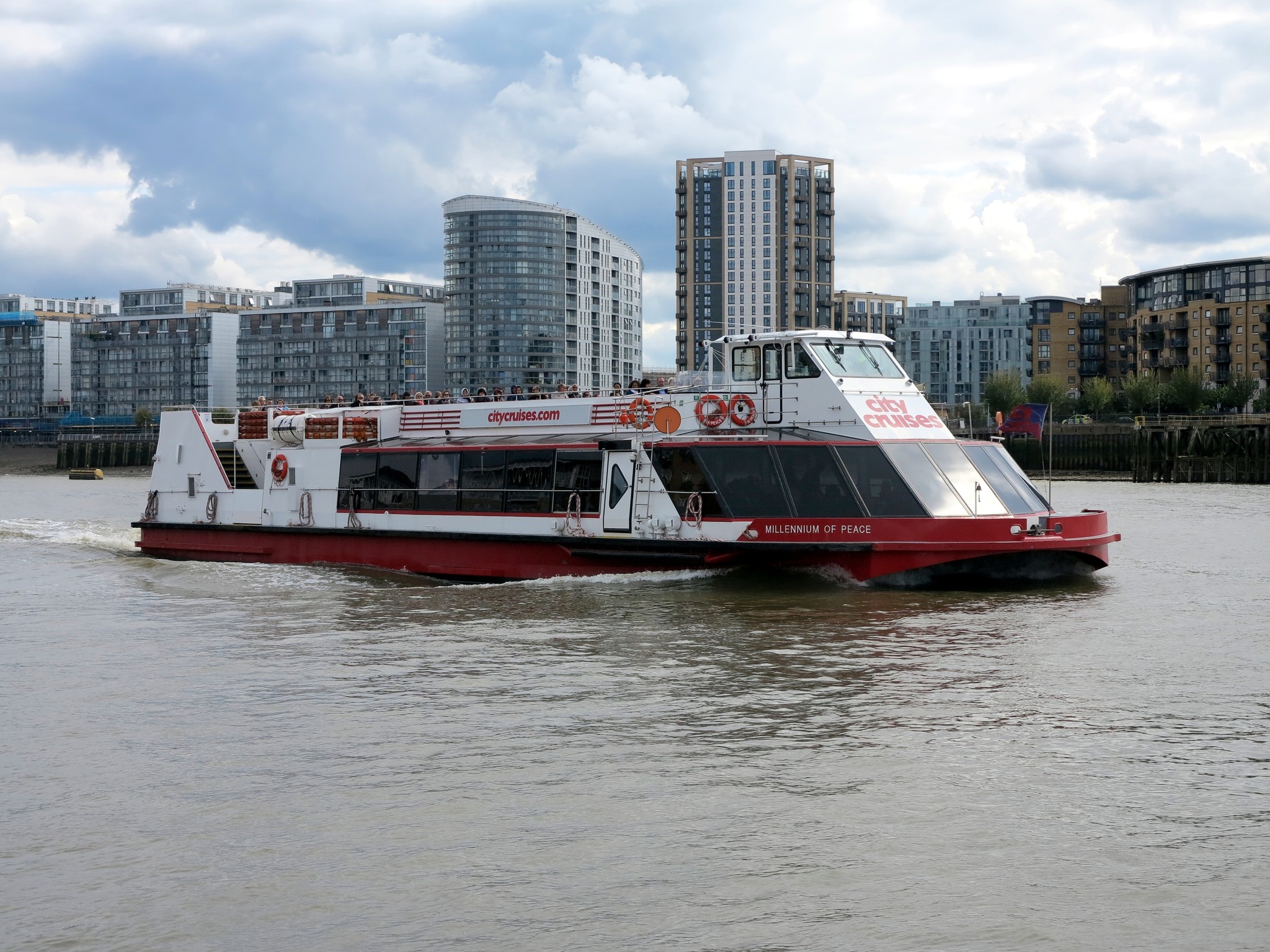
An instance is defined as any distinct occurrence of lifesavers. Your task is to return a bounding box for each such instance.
[271,454,288,481]
[695,395,728,427]
[628,398,654,430]
[728,394,756,426]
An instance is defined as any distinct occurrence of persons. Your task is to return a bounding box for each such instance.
[249,376,709,413]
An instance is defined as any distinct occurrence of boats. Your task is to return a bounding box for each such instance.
[129,328,1122,584]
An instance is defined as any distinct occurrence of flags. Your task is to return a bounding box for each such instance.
[997,403,1048,441]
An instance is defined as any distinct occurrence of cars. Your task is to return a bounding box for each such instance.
[1119,417,1134,422]
[1061,414,1093,425]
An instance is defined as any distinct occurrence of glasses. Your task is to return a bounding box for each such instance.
[425,395,431,397]
[532,389,537,391]
[357,397,364,399]
[326,399,331,401]
[695,380,702,382]
[657,381,664,383]
[671,382,677,384]
[416,395,422,397]
[405,396,410,398]
[337,399,343,401]
[444,394,449,396]
[437,394,442,395]
[584,394,589,396]
